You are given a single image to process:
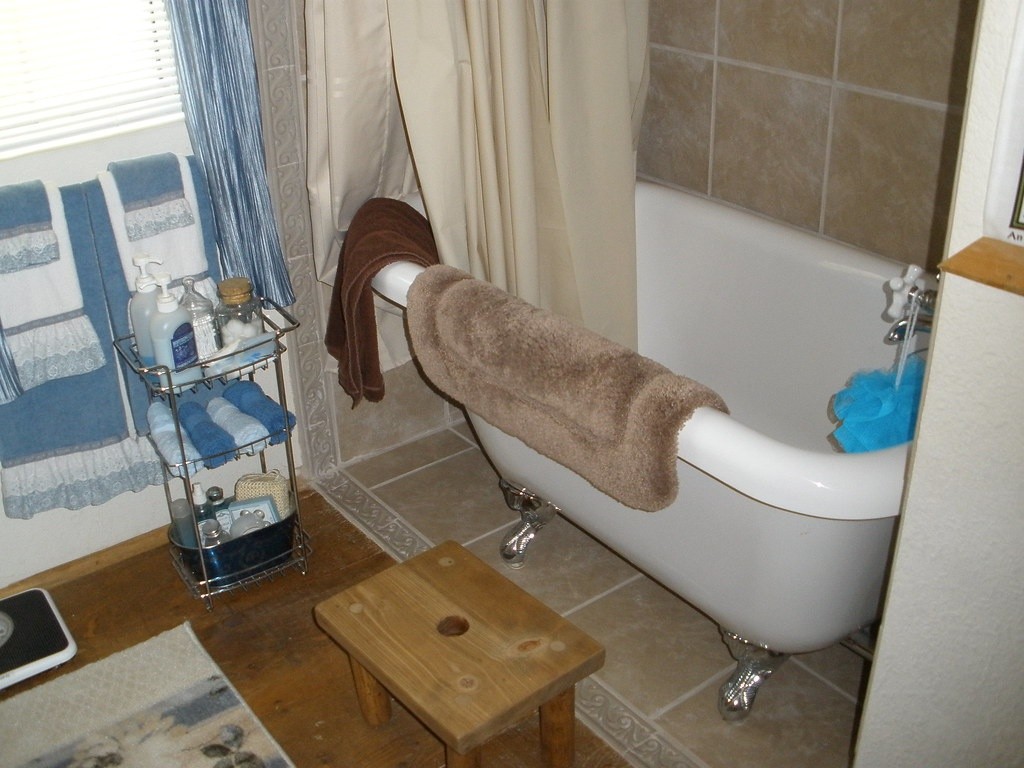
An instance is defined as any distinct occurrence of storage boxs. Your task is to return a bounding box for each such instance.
[166,504,295,585]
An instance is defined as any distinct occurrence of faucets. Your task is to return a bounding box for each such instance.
[888,313,934,343]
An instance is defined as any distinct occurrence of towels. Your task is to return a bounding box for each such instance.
[146,378,297,477]
[323,196,442,411]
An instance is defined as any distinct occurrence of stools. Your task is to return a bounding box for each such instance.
[313,540,606,768]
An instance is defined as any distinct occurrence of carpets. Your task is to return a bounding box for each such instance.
[0,621,294,768]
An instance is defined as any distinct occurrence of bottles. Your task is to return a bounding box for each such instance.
[180,279,216,359]
[201,519,229,547]
[218,278,265,350]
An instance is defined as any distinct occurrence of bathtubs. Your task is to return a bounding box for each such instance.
[370,179,939,655]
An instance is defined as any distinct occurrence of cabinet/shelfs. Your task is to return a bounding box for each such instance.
[112,295,302,610]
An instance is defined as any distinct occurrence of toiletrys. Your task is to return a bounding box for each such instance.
[129,254,223,388]
[169,482,272,548]
